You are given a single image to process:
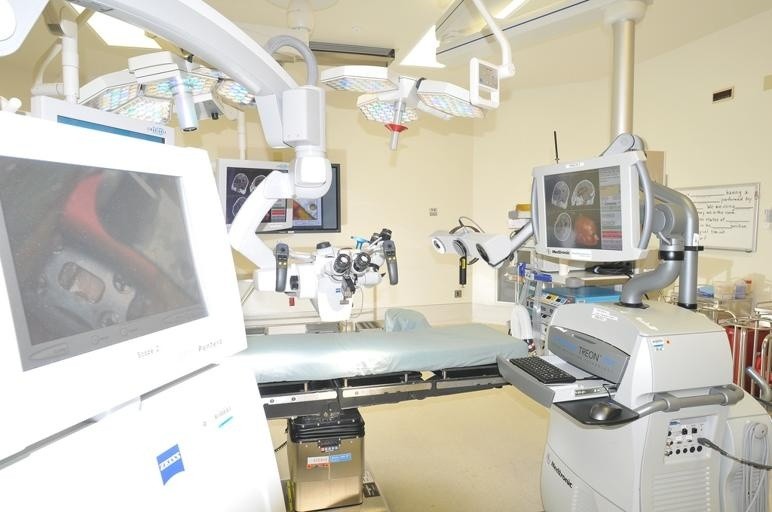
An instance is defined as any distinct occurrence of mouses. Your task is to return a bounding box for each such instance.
[590,402,624,421]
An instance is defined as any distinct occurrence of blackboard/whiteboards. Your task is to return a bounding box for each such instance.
[674,183,758,253]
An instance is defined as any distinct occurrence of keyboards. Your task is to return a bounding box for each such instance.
[510,356,576,384]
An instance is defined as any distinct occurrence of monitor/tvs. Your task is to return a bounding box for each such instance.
[0,114,247,461]
[531,152,652,262]
[218,159,293,232]
[31,95,175,145]
[292,164,342,233]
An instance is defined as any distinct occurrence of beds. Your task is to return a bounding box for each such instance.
[243,323,532,425]
